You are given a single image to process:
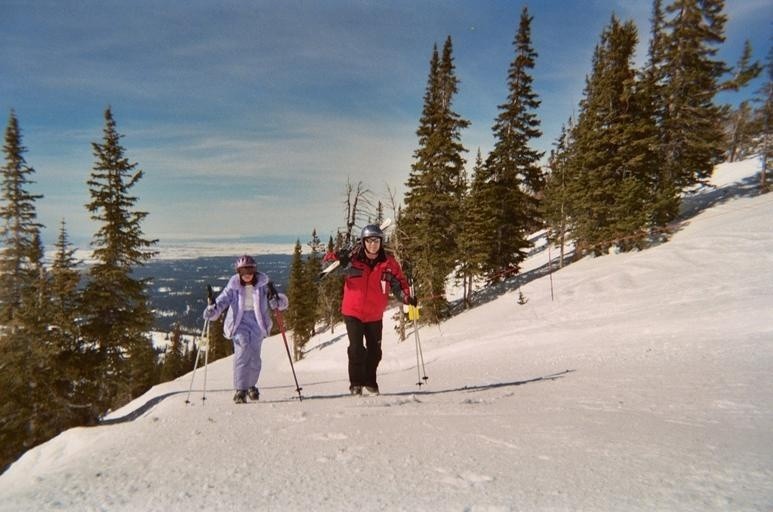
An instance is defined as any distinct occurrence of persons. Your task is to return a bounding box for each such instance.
[320,225,417,396]
[203,255,289,405]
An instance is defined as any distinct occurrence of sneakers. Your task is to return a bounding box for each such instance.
[247,386,259,401]
[350,384,363,396]
[365,382,379,393]
[232,390,247,404]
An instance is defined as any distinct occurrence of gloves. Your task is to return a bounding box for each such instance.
[335,250,350,267]
[410,296,418,308]
[206,304,216,317]
[268,294,280,310]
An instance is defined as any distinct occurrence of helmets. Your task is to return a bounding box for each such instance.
[234,255,257,274]
[361,224,384,250]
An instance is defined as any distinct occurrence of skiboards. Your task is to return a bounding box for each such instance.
[317,218,393,279]
